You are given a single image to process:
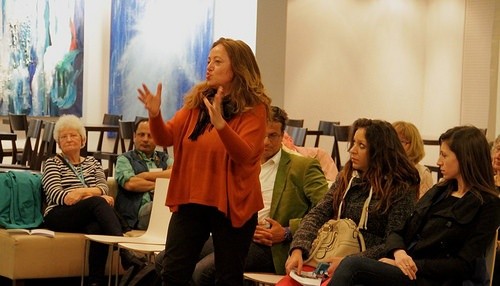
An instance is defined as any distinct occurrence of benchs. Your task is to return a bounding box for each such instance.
[0,174,161,286]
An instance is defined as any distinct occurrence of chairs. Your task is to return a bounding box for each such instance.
[421,140,444,184]
[244,270,286,286]
[81,177,175,286]
[0,113,166,174]
[287,120,354,173]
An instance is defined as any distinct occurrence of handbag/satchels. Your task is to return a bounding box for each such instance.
[300,218,368,267]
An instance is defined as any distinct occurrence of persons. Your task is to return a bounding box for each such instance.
[328,125,500,286]
[488,135,500,197]
[392,121,434,201]
[136,37,267,286]
[42,114,145,286]
[113,118,174,230]
[275,118,421,286]
[140,107,336,286]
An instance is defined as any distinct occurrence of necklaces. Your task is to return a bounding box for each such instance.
[61,153,91,188]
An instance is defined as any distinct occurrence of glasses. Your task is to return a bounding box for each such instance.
[262,132,283,142]
